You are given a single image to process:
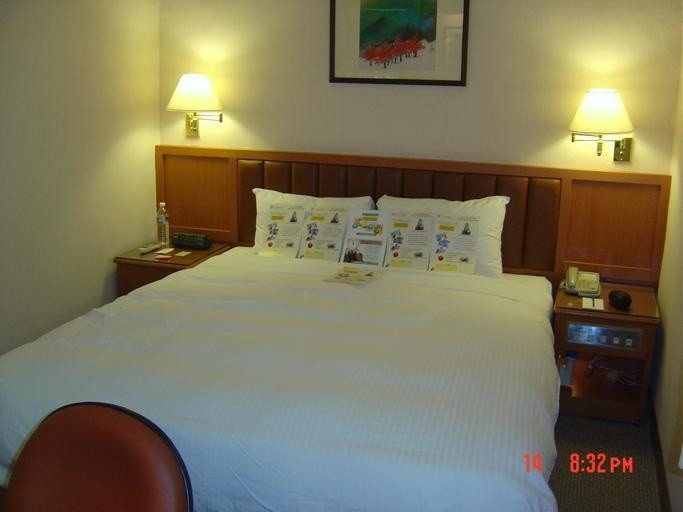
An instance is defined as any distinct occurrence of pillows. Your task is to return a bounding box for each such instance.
[247,186,509,278]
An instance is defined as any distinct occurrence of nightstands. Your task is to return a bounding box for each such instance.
[113,239,232,297]
[552,279,661,425]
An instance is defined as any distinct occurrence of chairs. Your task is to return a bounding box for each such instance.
[0,401,193,512]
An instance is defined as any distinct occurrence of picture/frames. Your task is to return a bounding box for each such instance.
[329,0,470,87]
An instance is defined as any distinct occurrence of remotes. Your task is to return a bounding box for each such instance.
[139,242,161,253]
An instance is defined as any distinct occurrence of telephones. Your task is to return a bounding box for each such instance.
[564,268,600,297]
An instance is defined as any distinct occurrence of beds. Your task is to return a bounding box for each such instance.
[0,244,572,510]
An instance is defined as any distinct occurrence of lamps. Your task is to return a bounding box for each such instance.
[568,88,634,161]
[166,73,223,138]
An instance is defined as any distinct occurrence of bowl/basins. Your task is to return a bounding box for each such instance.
[609,289,633,308]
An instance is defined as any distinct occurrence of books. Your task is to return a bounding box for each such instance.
[322,262,382,289]
[382,209,435,271]
[255,202,305,259]
[296,207,350,263]
[338,206,393,267]
[427,210,481,277]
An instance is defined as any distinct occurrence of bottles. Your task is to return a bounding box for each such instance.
[155,202,170,250]
